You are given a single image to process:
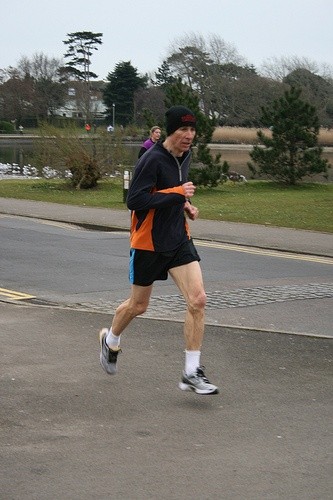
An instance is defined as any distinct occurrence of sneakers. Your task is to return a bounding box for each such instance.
[99,328,122,375]
[178,365,218,394]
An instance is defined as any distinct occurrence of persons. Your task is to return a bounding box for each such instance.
[99,106,219,395]
[138,126,161,159]
[19,125,24,135]
[106,125,113,131]
[85,124,91,134]
[93,124,97,133]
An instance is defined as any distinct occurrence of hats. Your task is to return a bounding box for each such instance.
[165,106,196,137]
